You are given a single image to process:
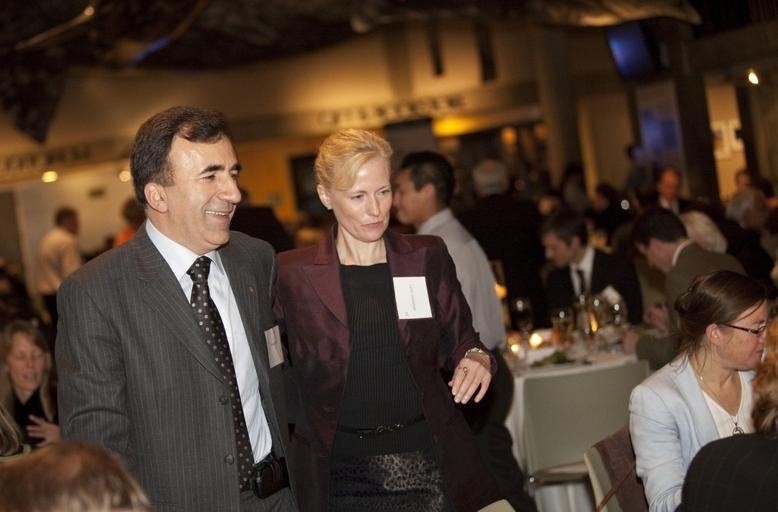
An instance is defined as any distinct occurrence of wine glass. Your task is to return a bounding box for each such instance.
[551,292,607,365]
[511,298,534,342]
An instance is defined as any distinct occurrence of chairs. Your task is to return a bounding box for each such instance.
[583,424,649,512]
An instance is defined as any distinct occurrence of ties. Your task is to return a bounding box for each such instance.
[578,271,586,295]
[187,256,254,491]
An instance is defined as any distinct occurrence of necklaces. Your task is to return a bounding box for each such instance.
[692,366,744,435]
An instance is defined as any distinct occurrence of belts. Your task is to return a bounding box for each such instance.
[339,416,423,436]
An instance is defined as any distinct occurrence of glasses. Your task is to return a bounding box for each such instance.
[723,323,767,334]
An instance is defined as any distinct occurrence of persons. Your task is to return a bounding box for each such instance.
[674,316,778,512]
[35,198,148,317]
[54,106,298,512]
[1,442,148,512]
[274,128,517,512]
[392,144,778,371]
[392,152,538,512]
[0,320,59,456]
[628,270,768,512]
[229,189,294,253]
